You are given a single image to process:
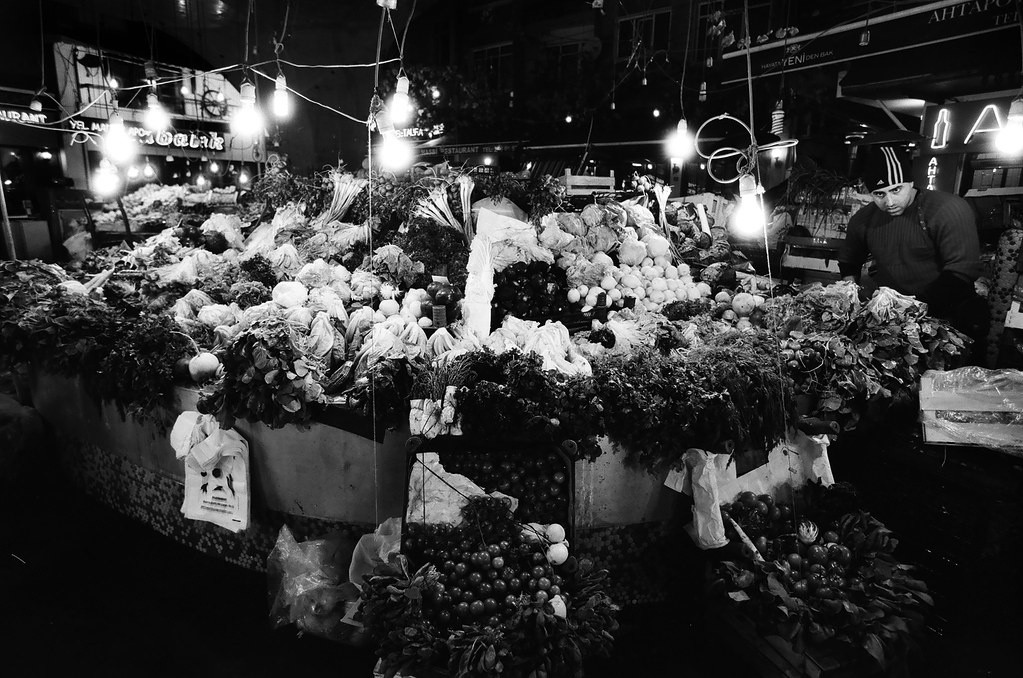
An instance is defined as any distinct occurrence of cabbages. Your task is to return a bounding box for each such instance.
[537,195,744,294]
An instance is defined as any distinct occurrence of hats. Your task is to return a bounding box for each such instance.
[856,145,914,193]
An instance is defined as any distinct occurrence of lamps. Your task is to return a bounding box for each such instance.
[770,96,786,135]
[860,30,871,47]
[29,101,43,112]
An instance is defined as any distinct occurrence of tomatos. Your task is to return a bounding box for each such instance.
[398,449,570,619]
[718,490,867,603]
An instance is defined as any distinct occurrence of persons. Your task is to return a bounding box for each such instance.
[838,142,991,369]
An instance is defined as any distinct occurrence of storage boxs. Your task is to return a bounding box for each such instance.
[15,344,832,527]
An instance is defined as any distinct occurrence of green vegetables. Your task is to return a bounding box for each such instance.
[577,277,974,464]
[0,165,603,443]
